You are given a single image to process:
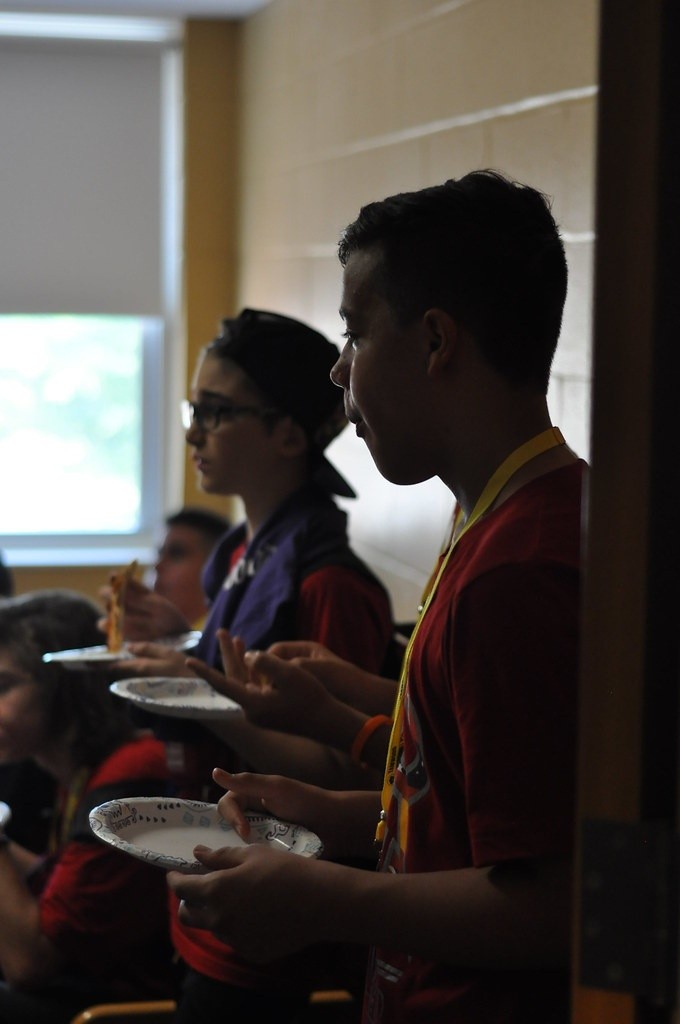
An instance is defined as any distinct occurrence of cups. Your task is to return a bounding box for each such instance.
[0,802,10,827]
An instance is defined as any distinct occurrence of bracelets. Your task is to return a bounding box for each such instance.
[350,710,391,762]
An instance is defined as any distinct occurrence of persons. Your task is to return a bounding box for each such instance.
[0,184,678,1020]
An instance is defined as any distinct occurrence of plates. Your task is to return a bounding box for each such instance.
[41,631,206,670]
[109,675,244,721]
[88,796,323,874]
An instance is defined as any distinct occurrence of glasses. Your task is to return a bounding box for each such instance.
[182,402,279,433]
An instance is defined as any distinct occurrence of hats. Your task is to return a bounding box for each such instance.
[224,309,358,498]
